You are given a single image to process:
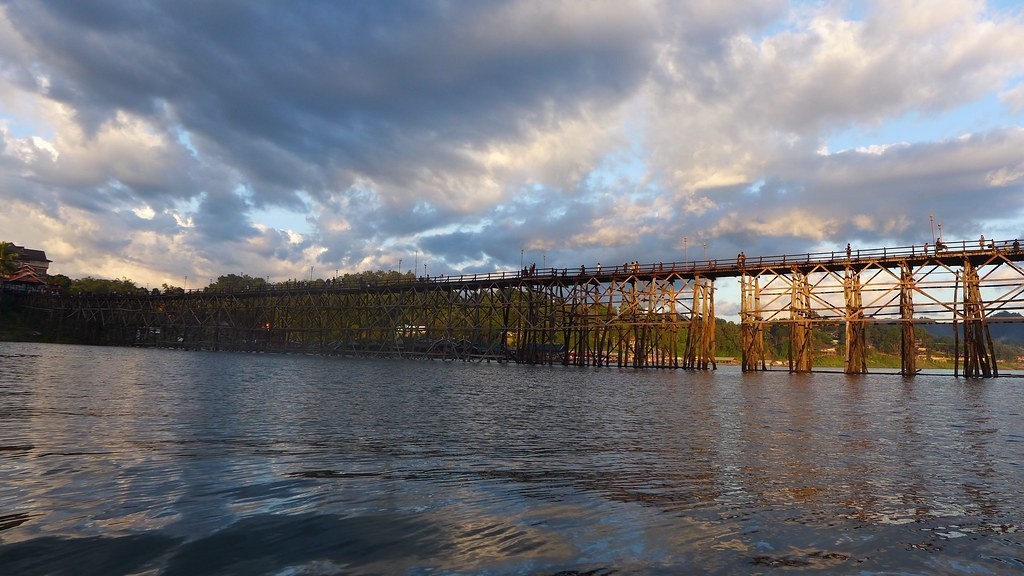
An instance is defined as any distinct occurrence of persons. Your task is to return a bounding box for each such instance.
[1004,242,1010,252]
[630,261,640,273]
[1013,239,1019,252]
[597,263,601,274]
[659,262,663,271]
[979,235,985,249]
[737,252,746,267]
[524,263,535,277]
[936,238,947,248]
[845,243,851,259]
[581,265,585,274]
[924,243,929,252]
